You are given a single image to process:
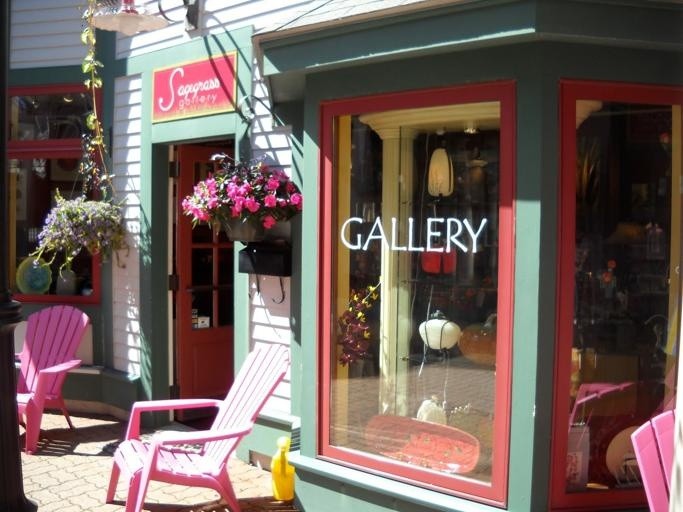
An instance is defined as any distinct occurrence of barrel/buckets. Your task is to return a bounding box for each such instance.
[565,424,590,492]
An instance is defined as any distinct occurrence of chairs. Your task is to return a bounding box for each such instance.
[105,342,289,512]
[14,303,91,454]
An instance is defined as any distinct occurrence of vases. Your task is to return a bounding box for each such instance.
[224,215,260,242]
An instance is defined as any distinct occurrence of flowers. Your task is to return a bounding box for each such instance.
[181,156,303,231]
[27,185,130,283]
